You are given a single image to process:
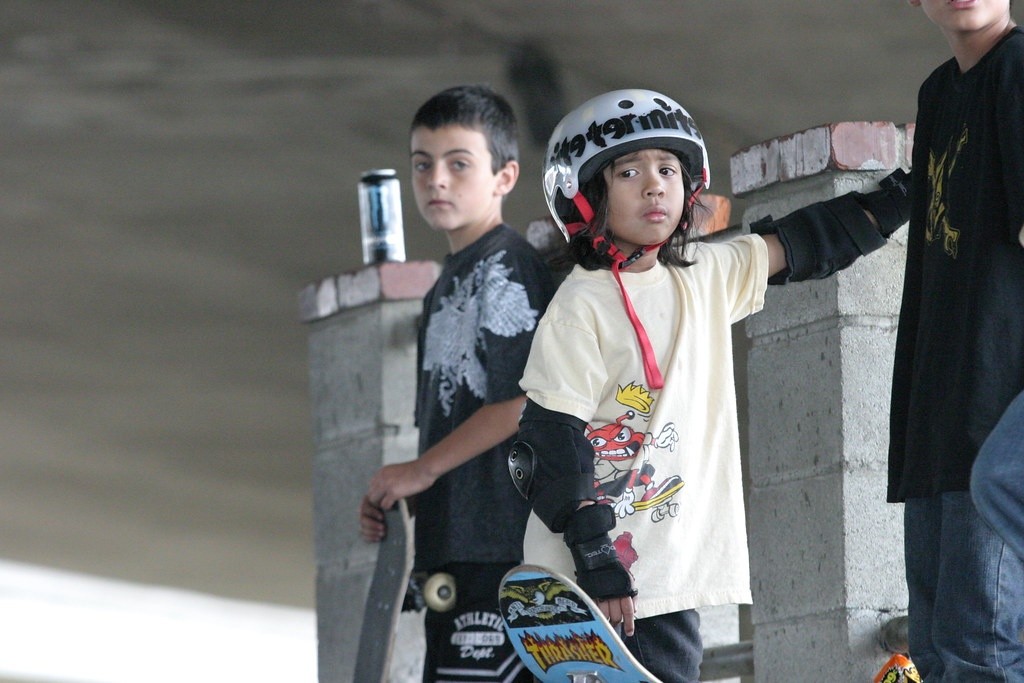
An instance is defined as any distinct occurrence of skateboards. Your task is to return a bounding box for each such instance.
[353,485,459,683]
[497,562,671,683]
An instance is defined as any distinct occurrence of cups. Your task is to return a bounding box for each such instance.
[356,167,408,263]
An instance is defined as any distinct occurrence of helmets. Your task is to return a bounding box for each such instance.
[542,89,711,246]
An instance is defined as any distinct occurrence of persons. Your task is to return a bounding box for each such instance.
[355,83,554,683]
[884,0,1024,683]
[508,90,914,683]
[970,391,1023,561]
